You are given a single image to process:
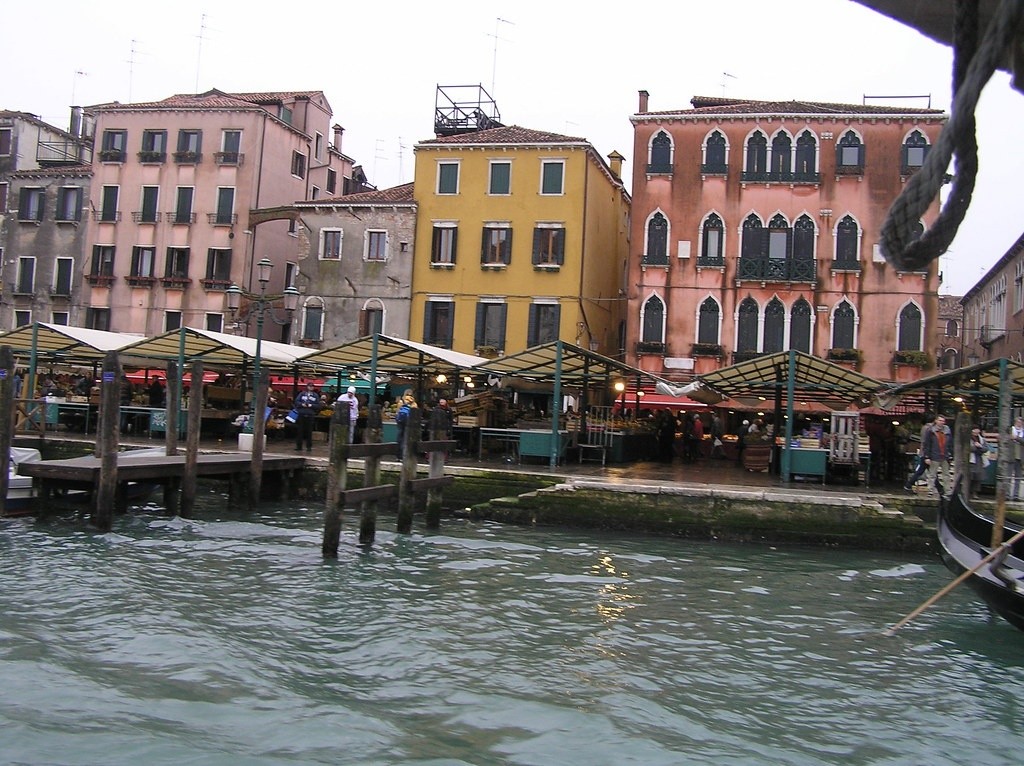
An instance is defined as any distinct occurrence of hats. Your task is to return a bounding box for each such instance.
[927,414,935,423]
[307,382,315,387]
[347,386,357,394]
[440,399,446,406]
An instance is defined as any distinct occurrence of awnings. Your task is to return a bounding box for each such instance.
[114,326,324,441]
[692,346,894,482]
[468,339,685,471]
[0,320,146,430]
[893,355,1024,482]
[294,331,490,441]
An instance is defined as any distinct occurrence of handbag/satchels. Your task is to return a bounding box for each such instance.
[714,437,723,447]
[446,427,453,436]
[286,410,299,423]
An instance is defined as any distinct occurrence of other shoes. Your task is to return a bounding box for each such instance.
[973,495,979,500]
[307,447,311,451]
[294,447,302,450]
[927,494,932,497]
[1004,497,1009,501]
[396,458,403,463]
[1013,498,1021,502]
[904,484,913,492]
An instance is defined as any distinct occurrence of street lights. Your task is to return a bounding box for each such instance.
[223,250,300,433]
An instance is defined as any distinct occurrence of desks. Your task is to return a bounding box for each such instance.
[479,427,570,467]
[381,421,425,443]
[449,425,479,456]
[782,447,872,491]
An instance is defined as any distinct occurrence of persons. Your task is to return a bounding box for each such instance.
[241,404,250,414]
[564,406,582,418]
[517,404,528,418]
[395,389,453,463]
[711,413,726,460]
[590,407,602,419]
[649,409,705,465]
[1012,417,1024,501]
[904,413,953,498]
[609,409,623,422]
[268,383,389,452]
[736,419,761,464]
[969,424,989,499]
[119,374,239,434]
[13,369,96,425]
[625,409,633,420]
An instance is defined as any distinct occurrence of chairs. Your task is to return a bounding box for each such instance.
[576,405,615,466]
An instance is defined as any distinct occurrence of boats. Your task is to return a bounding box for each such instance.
[935,475,1023,632]
[7,447,139,519]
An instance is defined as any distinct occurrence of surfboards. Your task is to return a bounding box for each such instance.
[884,532,1024,635]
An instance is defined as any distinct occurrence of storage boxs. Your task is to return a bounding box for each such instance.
[312,431,327,441]
[238,433,266,451]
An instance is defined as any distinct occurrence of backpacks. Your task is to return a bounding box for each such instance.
[395,399,412,423]
